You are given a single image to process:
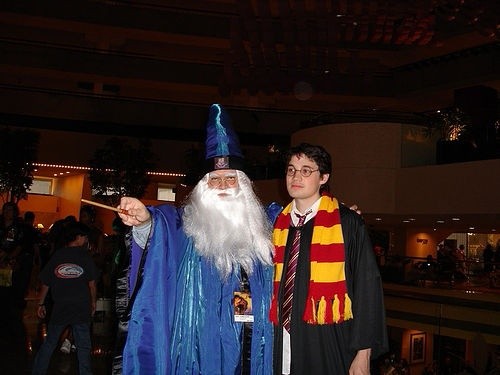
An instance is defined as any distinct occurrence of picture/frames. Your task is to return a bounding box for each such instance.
[410,333,427,364]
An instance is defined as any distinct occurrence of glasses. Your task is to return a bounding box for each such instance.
[284,167,319,177]
[207,177,237,186]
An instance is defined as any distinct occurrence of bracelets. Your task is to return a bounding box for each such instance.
[38,304,45,306]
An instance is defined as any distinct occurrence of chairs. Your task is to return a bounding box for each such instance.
[437,263,453,287]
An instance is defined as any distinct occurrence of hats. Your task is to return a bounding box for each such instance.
[202,104,249,174]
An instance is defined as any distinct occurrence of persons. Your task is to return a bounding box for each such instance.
[60,205,130,354]
[0,201,45,345]
[117,103,361,375]
[403,239,500,288]
[32,222,97,375]
[269,143,388,375]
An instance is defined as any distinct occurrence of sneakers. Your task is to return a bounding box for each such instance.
[60,339,71,353]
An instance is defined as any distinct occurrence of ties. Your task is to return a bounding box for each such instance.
[282,211,313,334]
